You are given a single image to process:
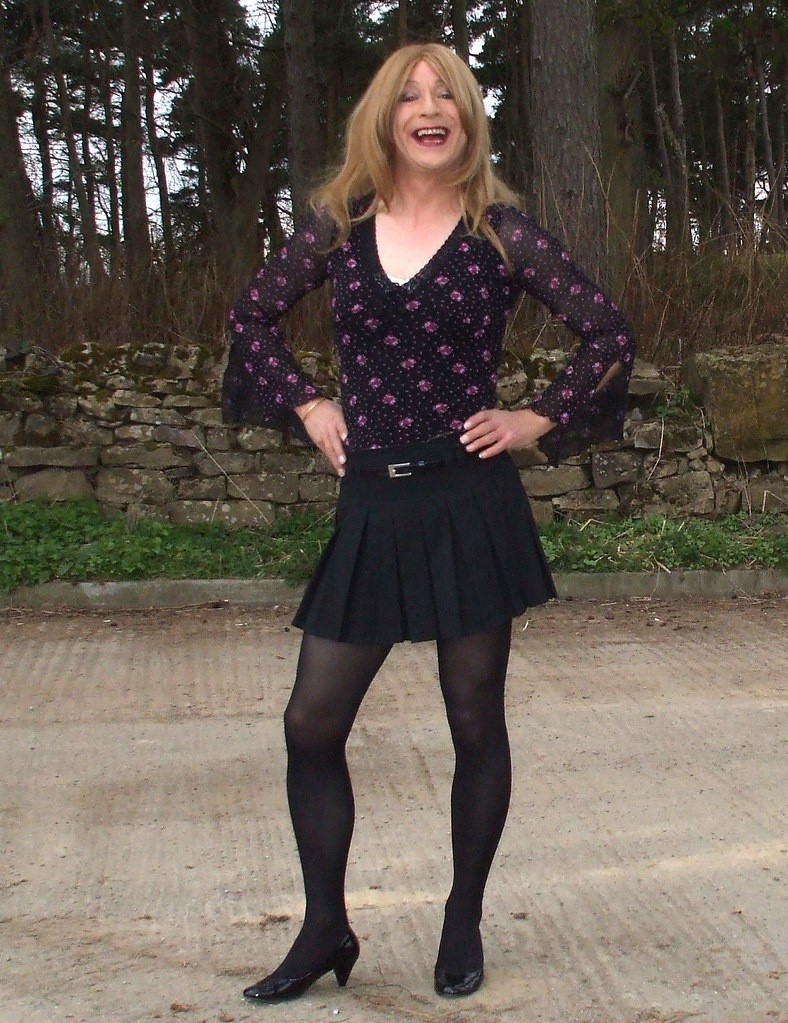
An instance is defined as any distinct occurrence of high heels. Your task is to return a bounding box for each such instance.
[243,927,359,1002]
[434,902,484,997]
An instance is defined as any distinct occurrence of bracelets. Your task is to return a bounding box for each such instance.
[303,397,326,424]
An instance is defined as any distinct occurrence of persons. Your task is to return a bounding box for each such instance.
[217,43,636,1001]
